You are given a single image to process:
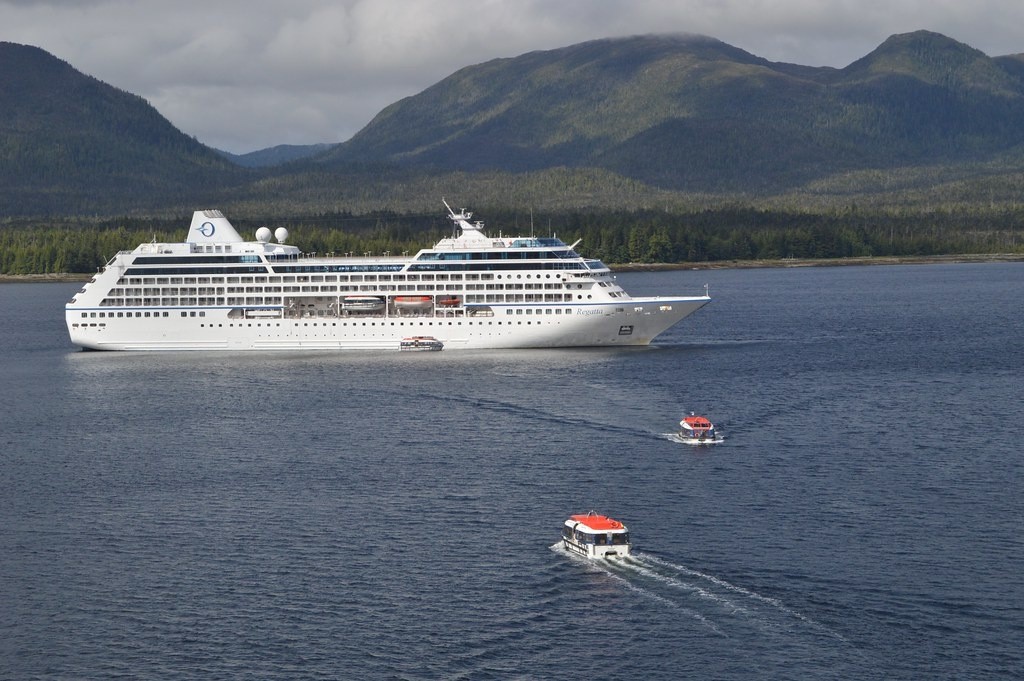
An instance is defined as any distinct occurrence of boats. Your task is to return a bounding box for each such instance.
[678,410,716,443]
[392,296,433,310]
[399,334,445,352]
[342,296,385,311]
[439,296,462,306]
[245,309,282,317]
[559,509,633,560]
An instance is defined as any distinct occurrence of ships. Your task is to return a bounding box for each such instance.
[62,194,714,354]
